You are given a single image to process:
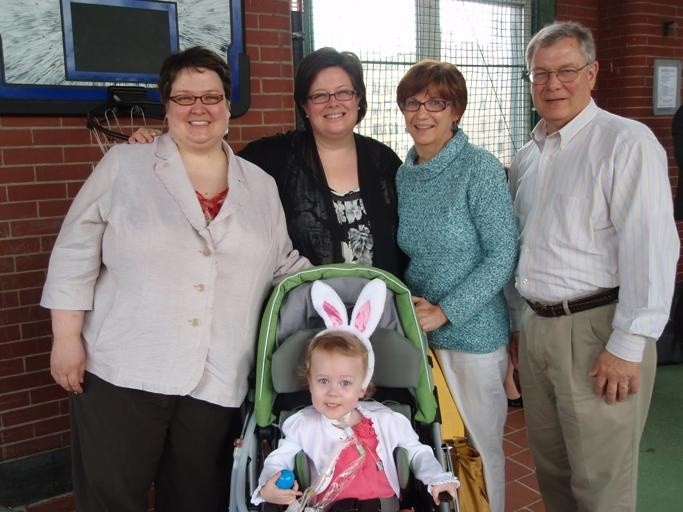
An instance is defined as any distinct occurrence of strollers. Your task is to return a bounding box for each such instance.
[225,261,463,511]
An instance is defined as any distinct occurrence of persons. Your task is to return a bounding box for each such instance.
[37,47,318,512]
[507,17,682,512]
[392,59,521,512]
[247,328,462,512]
[127,45,408,281]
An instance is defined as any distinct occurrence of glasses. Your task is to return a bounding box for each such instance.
[306,90,358,104]
[403,100,452,112]
[529,63,588,84]
[168,95,223,106]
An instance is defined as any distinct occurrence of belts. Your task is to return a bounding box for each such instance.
[523,287,619,317]
